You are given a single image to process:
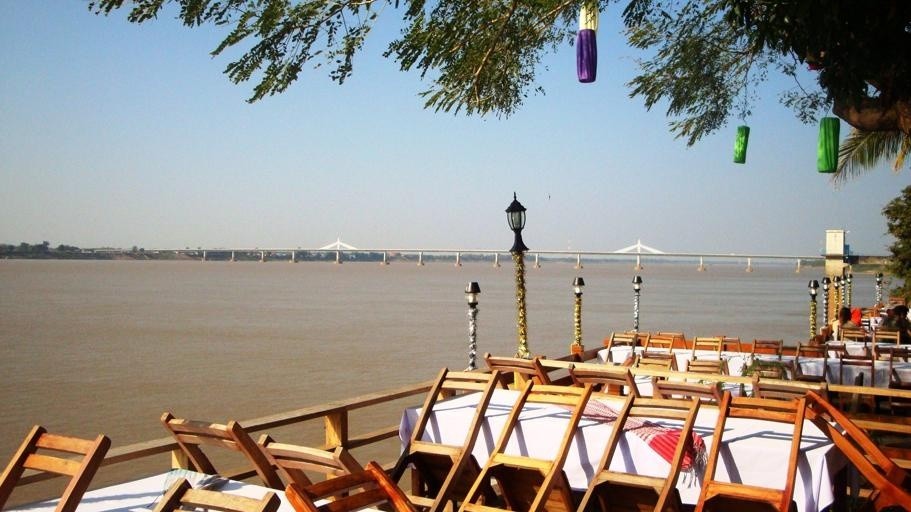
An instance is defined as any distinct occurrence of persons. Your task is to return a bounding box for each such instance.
[879,305,911,343]
[831,307,857,332]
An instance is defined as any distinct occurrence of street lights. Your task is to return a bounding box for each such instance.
[629,274,642,335]
[503,188,532,361]
[806,271,884,340]
[569,273,588,354]
[461,278,481,372]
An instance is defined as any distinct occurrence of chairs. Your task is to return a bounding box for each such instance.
[385,333,911,511]
[1,411,416,512]
[812,298,911,347]
